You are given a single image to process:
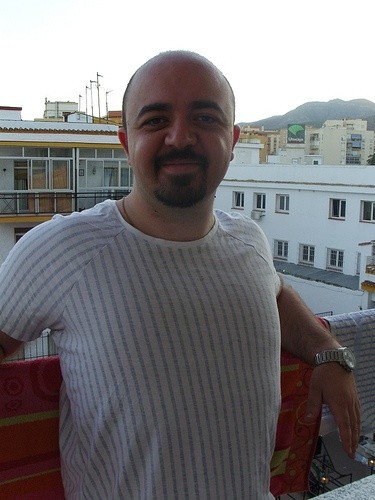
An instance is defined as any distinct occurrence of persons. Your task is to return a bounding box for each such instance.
[0,49,363,500]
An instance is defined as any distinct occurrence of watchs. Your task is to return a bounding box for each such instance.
[311,346,356,371]
